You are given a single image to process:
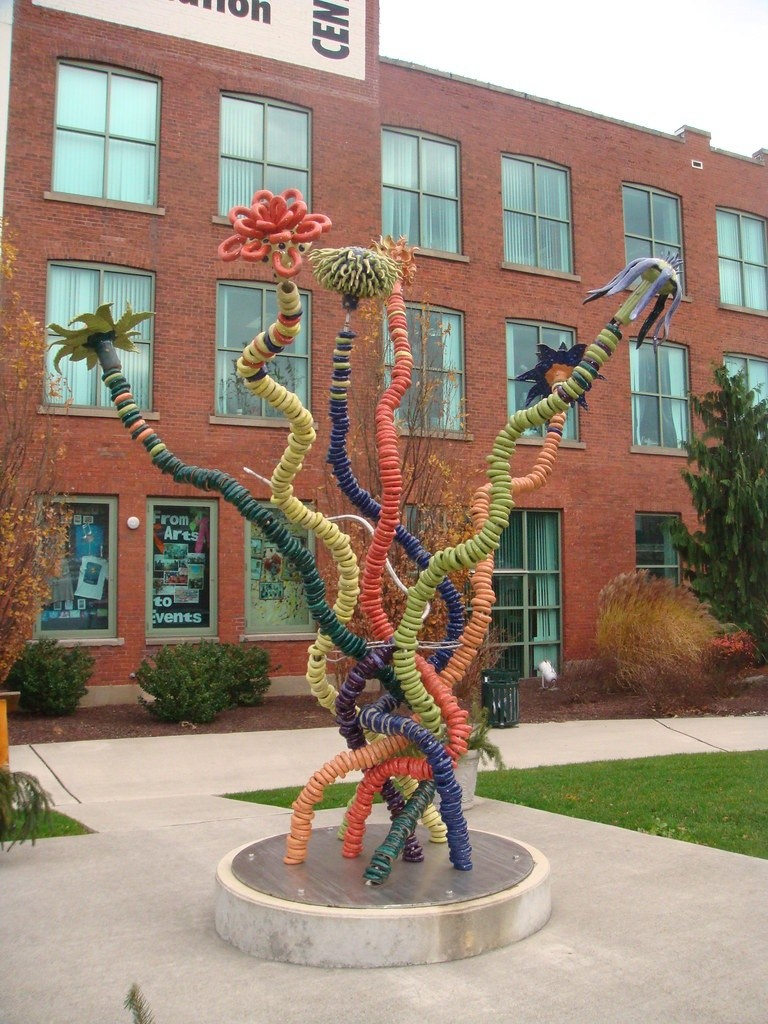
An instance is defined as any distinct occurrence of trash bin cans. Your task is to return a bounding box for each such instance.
[480,669,521,729]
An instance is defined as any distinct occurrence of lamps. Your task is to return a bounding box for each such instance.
[539,660,558,690]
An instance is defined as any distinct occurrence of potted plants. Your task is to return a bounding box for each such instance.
[435,681,504,812]
